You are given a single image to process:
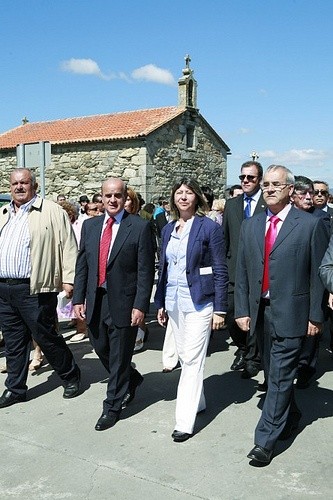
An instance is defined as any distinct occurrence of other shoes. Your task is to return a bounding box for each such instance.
[28,355,44,371]
[162,366,173,373]
[70,329,88,342]
[171,430,189,440]
[296,375,309,390]
[213,330,267,392]
[132,328,145,350]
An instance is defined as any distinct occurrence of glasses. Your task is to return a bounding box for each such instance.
[59,199,65,201]
[314,188,328,196]
[162,202,169,205]
[89,207,98,212]
[238,175,259,180]
[95,199,102,203]
[260,181,291,191]
[292,190,314,196]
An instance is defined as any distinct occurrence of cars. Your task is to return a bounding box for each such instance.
[0,193,13,208]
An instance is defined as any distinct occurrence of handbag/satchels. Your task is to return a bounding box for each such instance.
[57,290,87,318]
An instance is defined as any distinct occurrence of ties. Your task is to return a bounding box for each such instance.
[97,217,116,287]
[244,198,252,218]
[261,216,280,293]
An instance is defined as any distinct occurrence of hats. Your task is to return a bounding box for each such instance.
[78,196,90,203]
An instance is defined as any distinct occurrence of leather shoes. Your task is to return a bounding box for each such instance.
[63,364,81,398]
[278,411,302,442]
[247,444,272,462]
[95,414,119,431]
[121,374,144,406]
[0,389,26,408]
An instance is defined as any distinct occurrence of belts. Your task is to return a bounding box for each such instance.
[0,278,30,285]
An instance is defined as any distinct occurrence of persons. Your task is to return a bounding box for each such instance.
[0,168,85,409]
[73,177,156,430]
[233,164,327,464]
[154,176,228,442]
[0,161,333,380]
